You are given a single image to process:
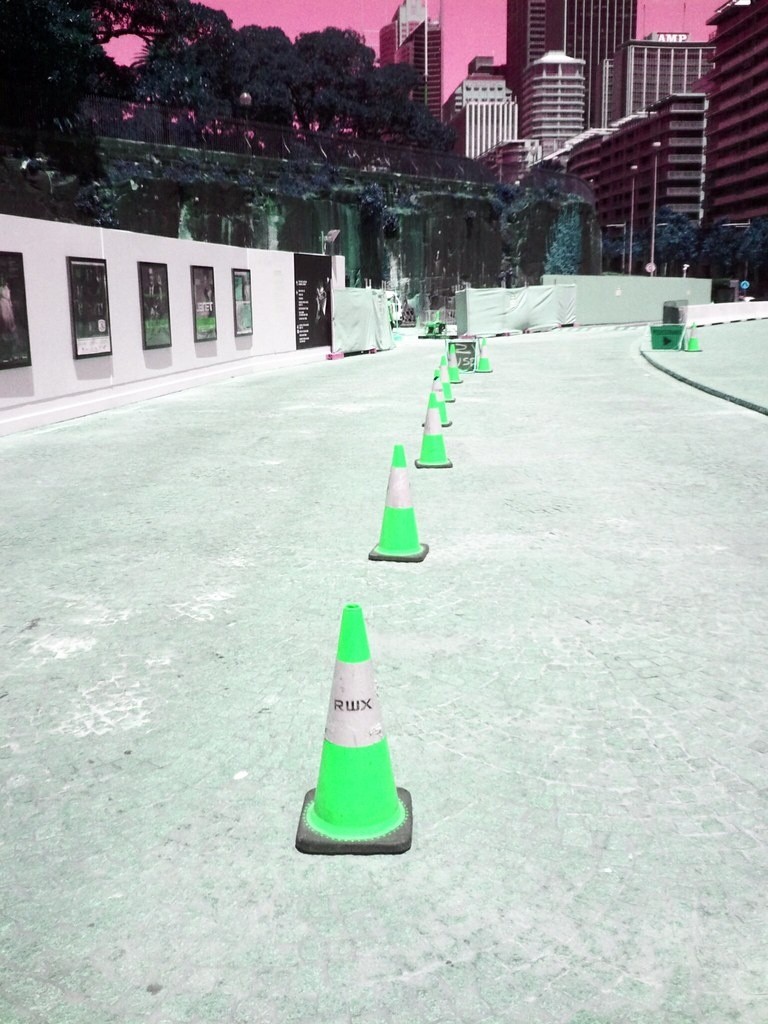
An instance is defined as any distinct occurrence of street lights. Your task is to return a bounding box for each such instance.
[651,140,661,276]
[628,165,637,276]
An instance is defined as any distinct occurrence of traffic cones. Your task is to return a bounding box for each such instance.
[421,369,453,428]
[296,603,414,858]
[683,322,704,353]
[447,344,465,384]
[474,338,493,373]
[414,392,455,470]
[368,443,428,564]
[436,356,456,404]
